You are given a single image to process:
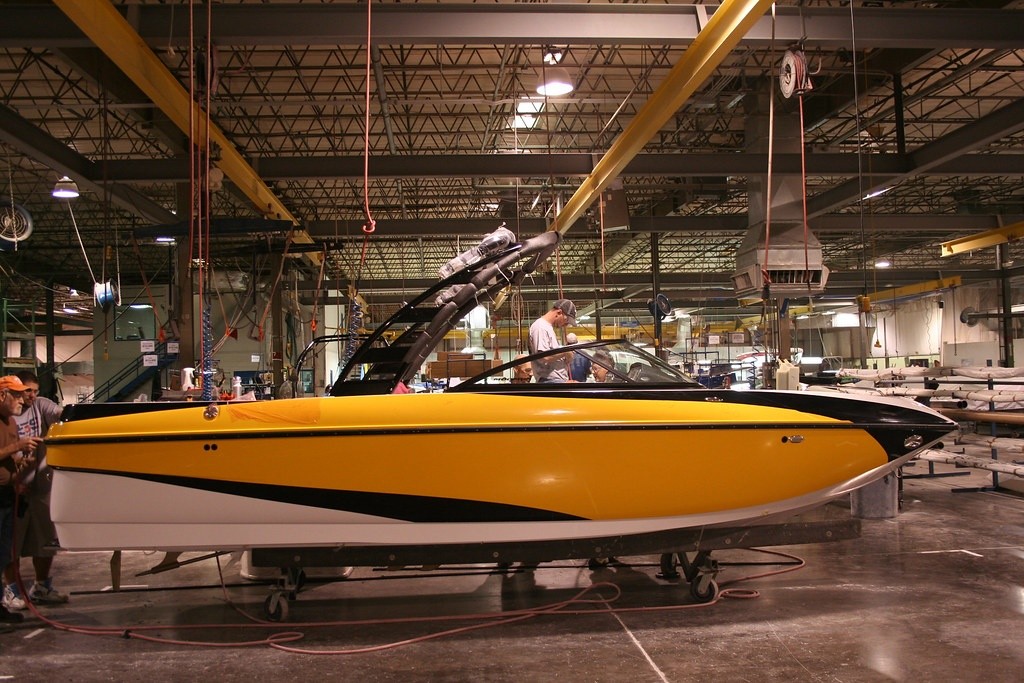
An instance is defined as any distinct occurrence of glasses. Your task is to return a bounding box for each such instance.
[6,391,23,398]
[590,366,601,372]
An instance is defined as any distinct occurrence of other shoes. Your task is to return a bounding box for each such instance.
[0,606,23,623]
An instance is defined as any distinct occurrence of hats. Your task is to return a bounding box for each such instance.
[553,299,578,327]
[566,333,577,343]
[0,375,31,392]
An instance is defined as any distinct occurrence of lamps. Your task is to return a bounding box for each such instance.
[53,176,79,198]
[536,49,574,96]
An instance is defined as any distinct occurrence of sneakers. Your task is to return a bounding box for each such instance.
[0,585,26,610]
[28,577,69,602]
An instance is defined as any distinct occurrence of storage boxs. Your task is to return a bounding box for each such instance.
[492,359,504,376]
[425,352,491,378]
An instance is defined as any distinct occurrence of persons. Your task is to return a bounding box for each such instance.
[528,299,578,383]
[566,332,592,383]
[386,374,410,394]
[0,375,44,622]
[2,371,69,609]
[510,354,533,384]
[591,347,625,383]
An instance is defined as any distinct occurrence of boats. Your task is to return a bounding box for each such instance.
[44,226,964,554]
